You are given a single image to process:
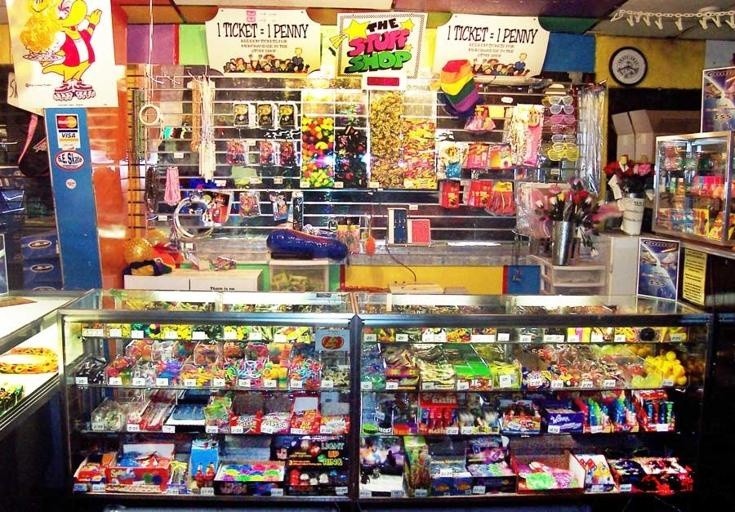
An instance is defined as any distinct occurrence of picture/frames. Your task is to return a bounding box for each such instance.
[699,66,735,133]
[635,238,681,304]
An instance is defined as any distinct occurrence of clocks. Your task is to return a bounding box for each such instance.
[609,46,648,88]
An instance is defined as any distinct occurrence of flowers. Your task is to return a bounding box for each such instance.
[535,183,605,259]
[603,154,655,194]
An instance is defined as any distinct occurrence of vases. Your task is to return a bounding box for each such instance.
[617,193,646,236]
[551,220,582,267]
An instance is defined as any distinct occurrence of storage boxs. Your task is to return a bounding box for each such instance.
[610,109,701,166]
